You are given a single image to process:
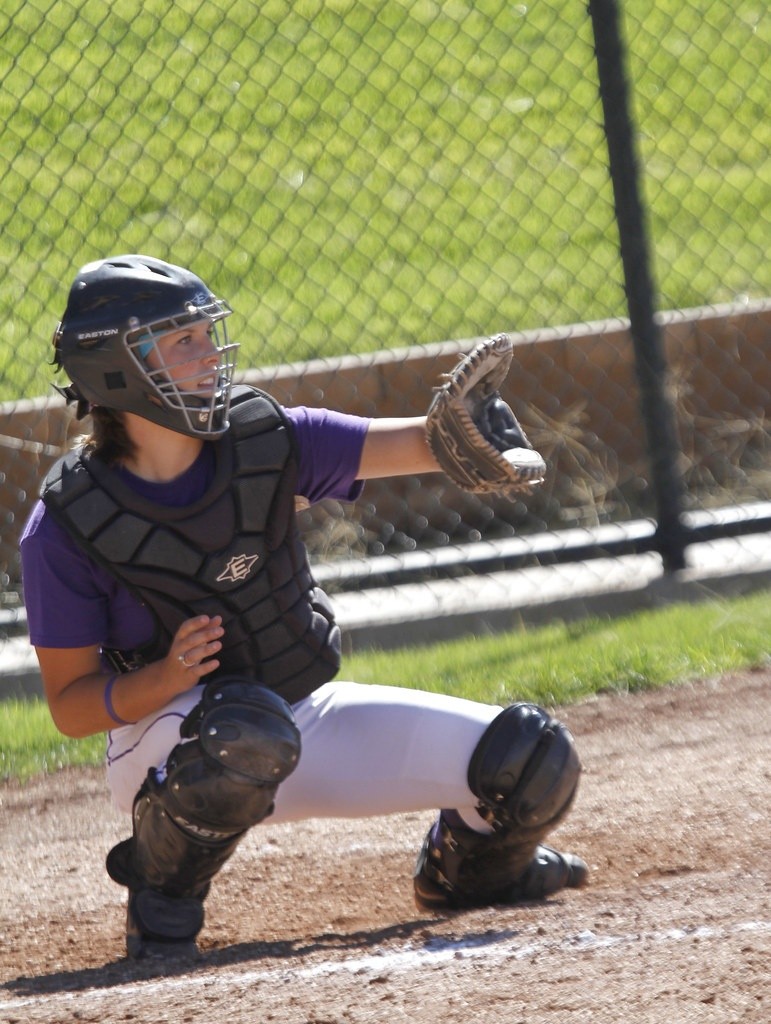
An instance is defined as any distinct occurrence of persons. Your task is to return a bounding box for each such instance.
[16,252,593,971]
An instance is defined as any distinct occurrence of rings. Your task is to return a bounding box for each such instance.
[178,656,196,667]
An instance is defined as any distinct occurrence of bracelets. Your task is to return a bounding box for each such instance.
[105,675,137,725]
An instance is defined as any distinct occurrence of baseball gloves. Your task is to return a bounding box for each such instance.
[425,331,549,502]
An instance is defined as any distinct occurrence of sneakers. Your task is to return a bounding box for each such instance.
[413,847,588,915]
[124,884,201,969]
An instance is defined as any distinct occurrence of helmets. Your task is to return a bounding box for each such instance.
[50,257,237,438]
